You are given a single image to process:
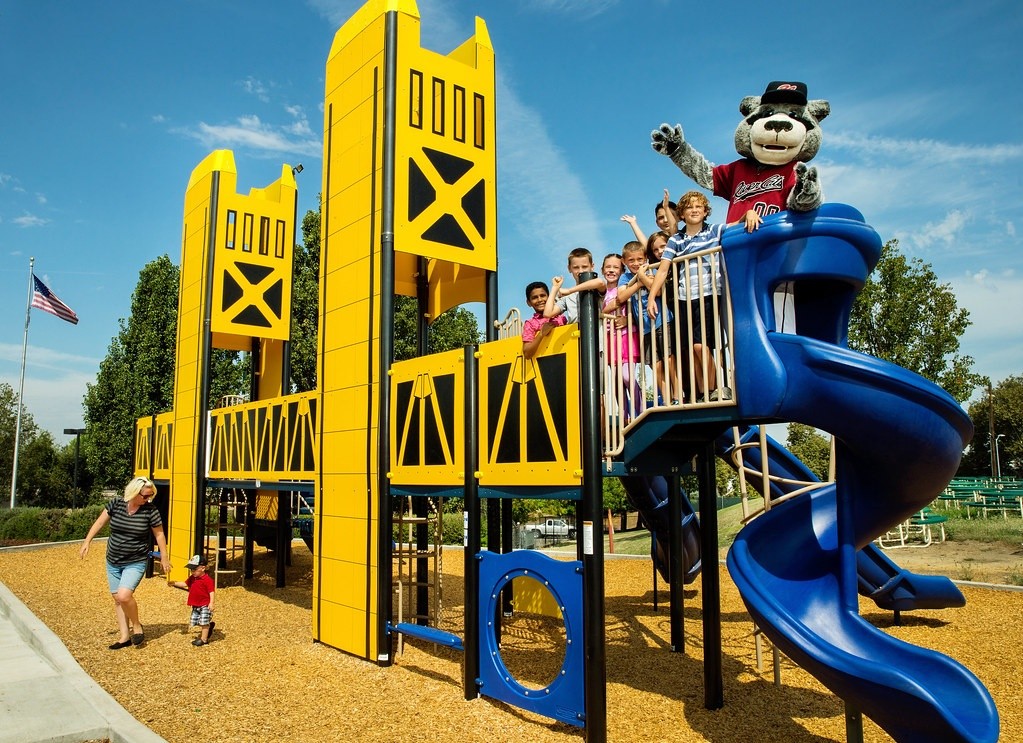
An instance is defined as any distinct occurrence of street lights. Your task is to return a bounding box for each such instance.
[64,428,91,534]
[994,434,1007,521]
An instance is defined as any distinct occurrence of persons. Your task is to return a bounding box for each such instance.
[522,191,763,449]
[166,555,215,645]
[79,477,173,648]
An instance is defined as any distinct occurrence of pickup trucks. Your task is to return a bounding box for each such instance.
[525,519,577,540]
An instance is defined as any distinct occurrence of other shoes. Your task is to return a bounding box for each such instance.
[109,637,131,649]
[133,625,143,645]
[192,638,208,646]
[207,622,215,638]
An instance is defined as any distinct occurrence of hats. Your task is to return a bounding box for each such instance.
[184,555,207,569]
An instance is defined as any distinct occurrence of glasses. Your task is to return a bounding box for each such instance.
[139,493,152,499]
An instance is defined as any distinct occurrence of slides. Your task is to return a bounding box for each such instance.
[618,472,703,585]
[718,198,1002,743]
[717,423,969,612]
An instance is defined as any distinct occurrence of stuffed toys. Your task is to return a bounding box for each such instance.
[652,81,830,401]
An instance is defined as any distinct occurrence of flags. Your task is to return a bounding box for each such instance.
[32,274,79,325]
